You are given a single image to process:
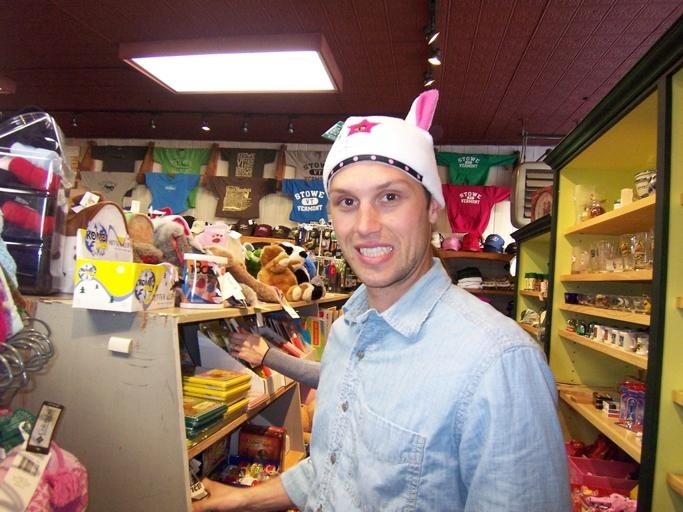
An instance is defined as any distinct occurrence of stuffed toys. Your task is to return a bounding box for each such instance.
[55,191,327,310]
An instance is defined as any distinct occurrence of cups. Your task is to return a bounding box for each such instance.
[179,253,228,309]
[564,231,654,356]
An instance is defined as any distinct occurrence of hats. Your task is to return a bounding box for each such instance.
[322,88,447,210]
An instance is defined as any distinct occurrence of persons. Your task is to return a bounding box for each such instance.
[227,325,321,390]
[190,114,572,512]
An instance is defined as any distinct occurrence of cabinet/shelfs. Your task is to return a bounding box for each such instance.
[433,249,516,296]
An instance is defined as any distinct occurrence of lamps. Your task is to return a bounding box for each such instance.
[118,32,343,97]
[148,112,295,137]
[423,25,441,87]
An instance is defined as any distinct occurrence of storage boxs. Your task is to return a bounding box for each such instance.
[0,112,78,293]
